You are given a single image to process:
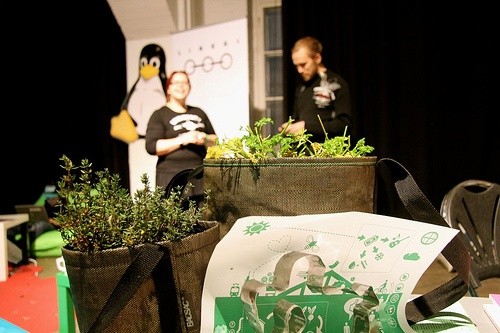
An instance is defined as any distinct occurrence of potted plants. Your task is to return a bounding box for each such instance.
[53,155,221,333]
[202,115,377,242]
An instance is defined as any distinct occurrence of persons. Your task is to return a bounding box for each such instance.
[145,70,219,209]
[277,37,350,152]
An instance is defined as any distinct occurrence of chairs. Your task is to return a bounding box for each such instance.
[440,180,500,297]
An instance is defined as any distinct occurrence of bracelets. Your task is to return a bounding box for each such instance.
[204,133,208,146]
